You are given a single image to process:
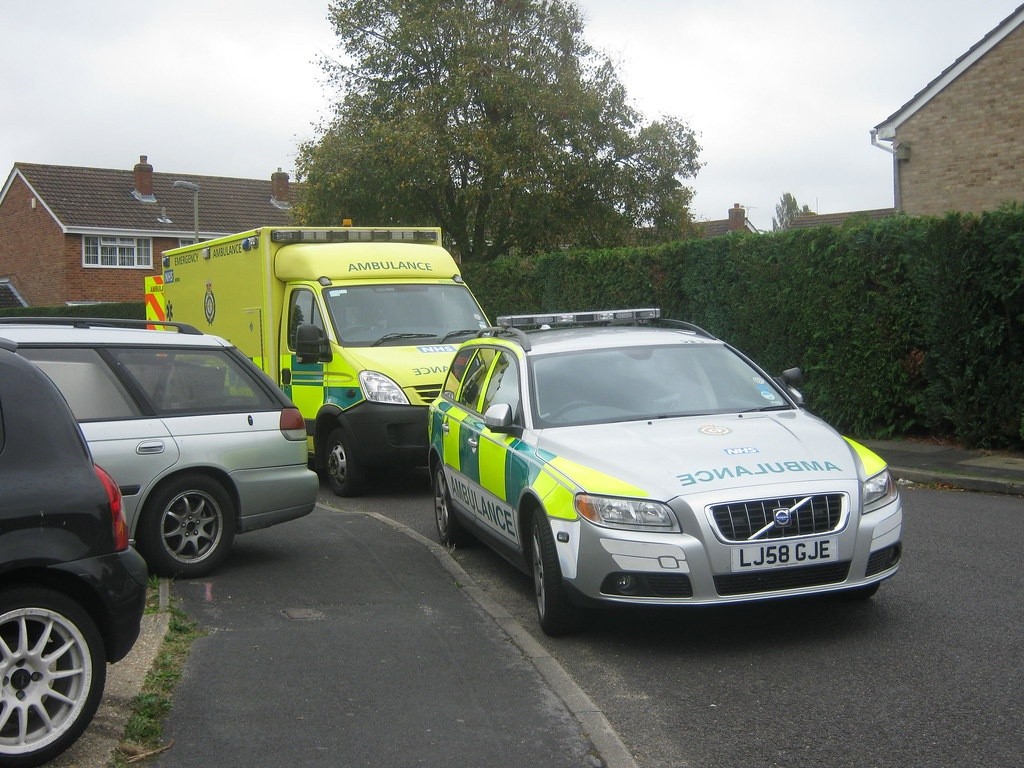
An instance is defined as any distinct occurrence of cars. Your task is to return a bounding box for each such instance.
[425,309,903,637]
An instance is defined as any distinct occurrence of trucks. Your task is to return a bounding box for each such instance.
[162,218,496,497]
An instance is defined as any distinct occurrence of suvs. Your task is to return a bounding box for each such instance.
[0,316,320,580]
[0,348,148,768]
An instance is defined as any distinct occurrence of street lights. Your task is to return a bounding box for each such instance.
[174,181,199,244]
